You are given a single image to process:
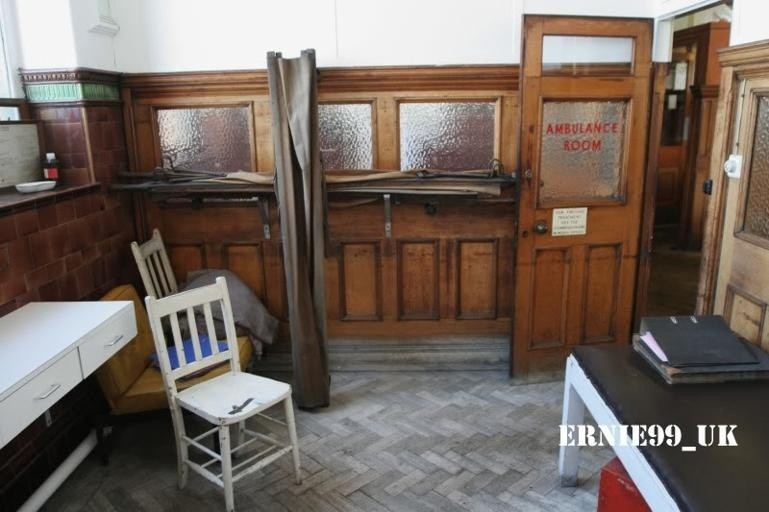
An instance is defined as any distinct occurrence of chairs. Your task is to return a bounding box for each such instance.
[130,229,248,337]
[94,284,254,466]
[144,275,303,511]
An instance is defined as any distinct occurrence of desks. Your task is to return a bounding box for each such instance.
[558,334,768,512]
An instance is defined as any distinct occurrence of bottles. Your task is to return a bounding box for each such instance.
[43,153,60,184]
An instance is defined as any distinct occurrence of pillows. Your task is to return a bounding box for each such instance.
[147,334,228,378]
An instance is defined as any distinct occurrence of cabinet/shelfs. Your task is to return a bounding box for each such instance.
[0,300,139,464]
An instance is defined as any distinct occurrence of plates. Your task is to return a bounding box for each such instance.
[14,180,56,193]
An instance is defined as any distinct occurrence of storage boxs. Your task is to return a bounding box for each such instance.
[597,456,651,512]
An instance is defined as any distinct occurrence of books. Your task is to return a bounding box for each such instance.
[632,314,769,386]
[148,336,230,380]
